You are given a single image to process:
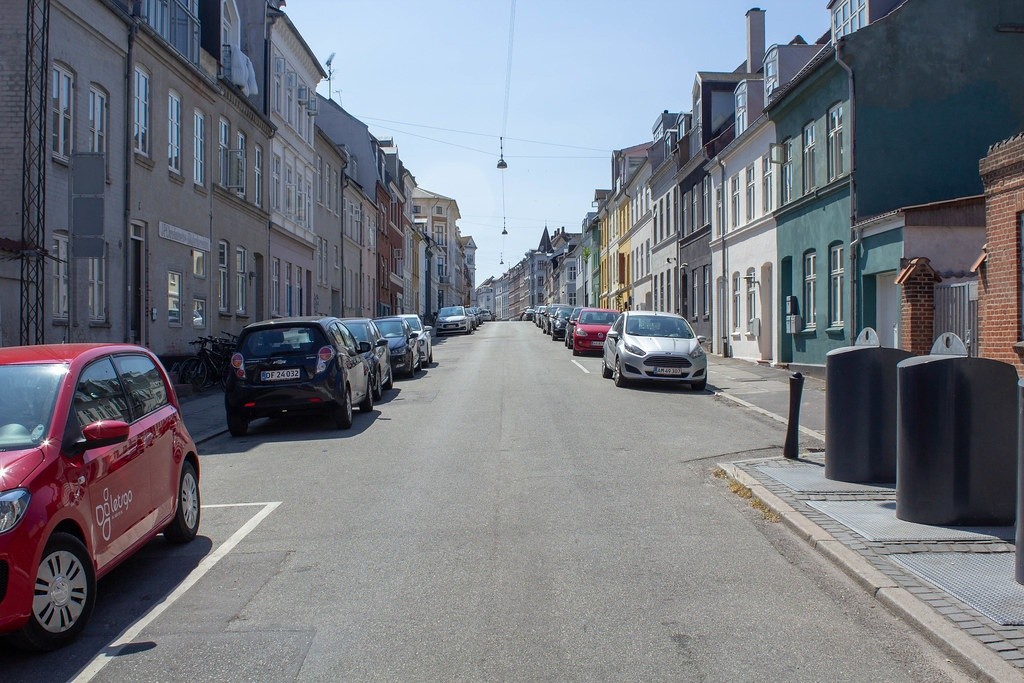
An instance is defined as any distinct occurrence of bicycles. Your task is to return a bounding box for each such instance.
[179,330,239,394]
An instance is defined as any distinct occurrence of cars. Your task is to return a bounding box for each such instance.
[433,305,473,337]
[520,303,599,349]
[570,307,624,356]
[0,343,201,655]
[367,317,423,379]
[601,310,708,391]
[225,315,374,437]
[462,305,493,331]
[295,317,393,401]
[387,312,433,369]
